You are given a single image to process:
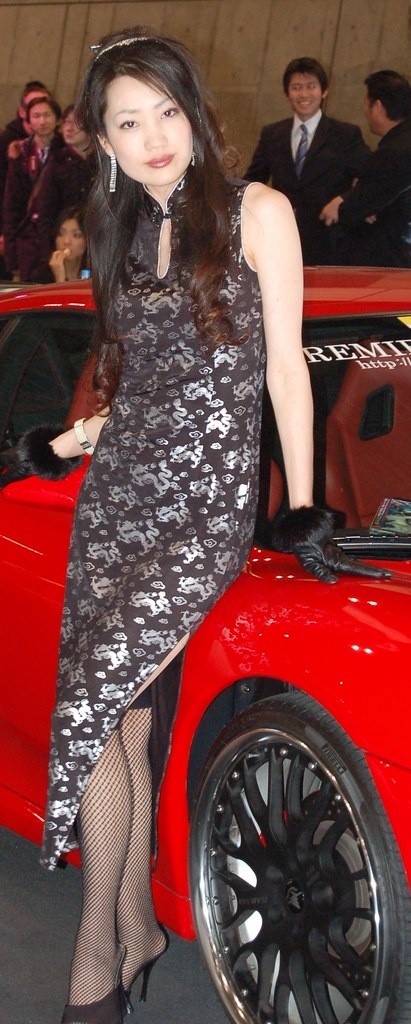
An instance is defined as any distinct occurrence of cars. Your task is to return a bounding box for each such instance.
[0,264,411,1023]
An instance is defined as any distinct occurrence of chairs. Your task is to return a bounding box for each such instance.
[311,334,411,530]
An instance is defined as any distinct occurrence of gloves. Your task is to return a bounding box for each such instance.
[269,503,394,584]
[0,423,83,491]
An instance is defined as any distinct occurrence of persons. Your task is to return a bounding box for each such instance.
[324,65,411,272]
[228,57,373,270]
[0,58,112,343]
[0,22,386,1024]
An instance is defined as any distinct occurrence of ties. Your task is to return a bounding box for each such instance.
[294,124,308,181]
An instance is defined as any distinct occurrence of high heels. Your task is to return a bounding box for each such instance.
[61,943,134,1024]
[121,945,168,1017]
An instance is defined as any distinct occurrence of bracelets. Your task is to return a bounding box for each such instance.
[74,417,95,456]
